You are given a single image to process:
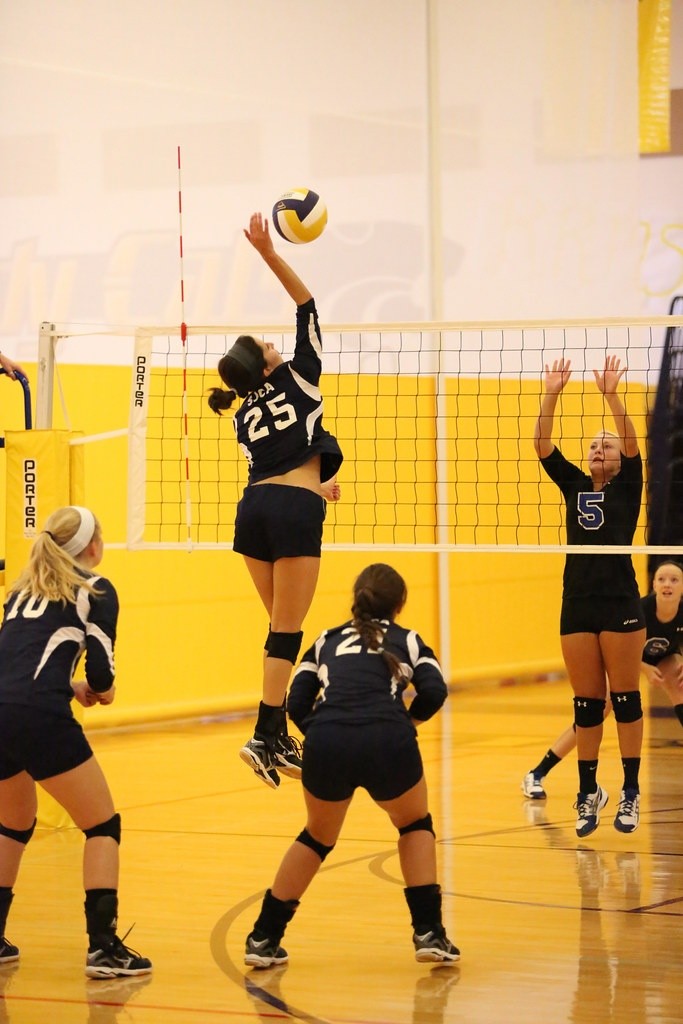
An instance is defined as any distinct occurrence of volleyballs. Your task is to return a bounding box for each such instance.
[271,188,329,246]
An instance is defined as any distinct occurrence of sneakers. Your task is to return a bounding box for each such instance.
[520,770,546,799]
[573,784,609,837]
[614,789,640,833]
[1,936,19,963]
[413,927,460,963]
[244,933,288,967]
[272,734,302,780]
[239,738,280,790]
[85,920,153,979]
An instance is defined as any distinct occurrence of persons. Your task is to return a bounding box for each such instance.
[535,354,646,837]
[0,507,153,979]
[520,560,683,800]
[208,211,343,791]
[244,562,460,967]
